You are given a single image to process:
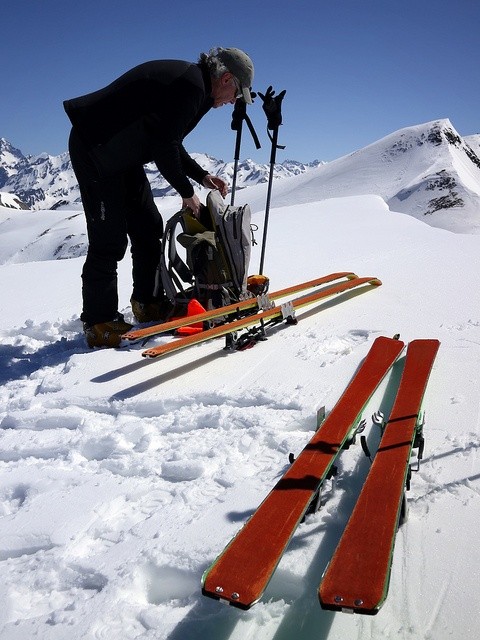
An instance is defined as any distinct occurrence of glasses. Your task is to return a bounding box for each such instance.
[234,80,242,99]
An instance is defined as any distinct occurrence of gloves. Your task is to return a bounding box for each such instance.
[231,91,256,131]
[257,87,286,130]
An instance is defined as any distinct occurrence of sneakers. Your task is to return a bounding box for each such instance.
[86,322,151,350]
[129,302,174,320]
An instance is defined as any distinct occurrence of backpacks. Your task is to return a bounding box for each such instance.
[153,191,257,327]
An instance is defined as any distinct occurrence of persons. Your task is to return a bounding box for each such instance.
[62,45,254,350]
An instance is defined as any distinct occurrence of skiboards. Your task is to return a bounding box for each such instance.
[121,272,381,359]
[201,335,441,615]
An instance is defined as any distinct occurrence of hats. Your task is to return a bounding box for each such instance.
[218,47,254,103]
